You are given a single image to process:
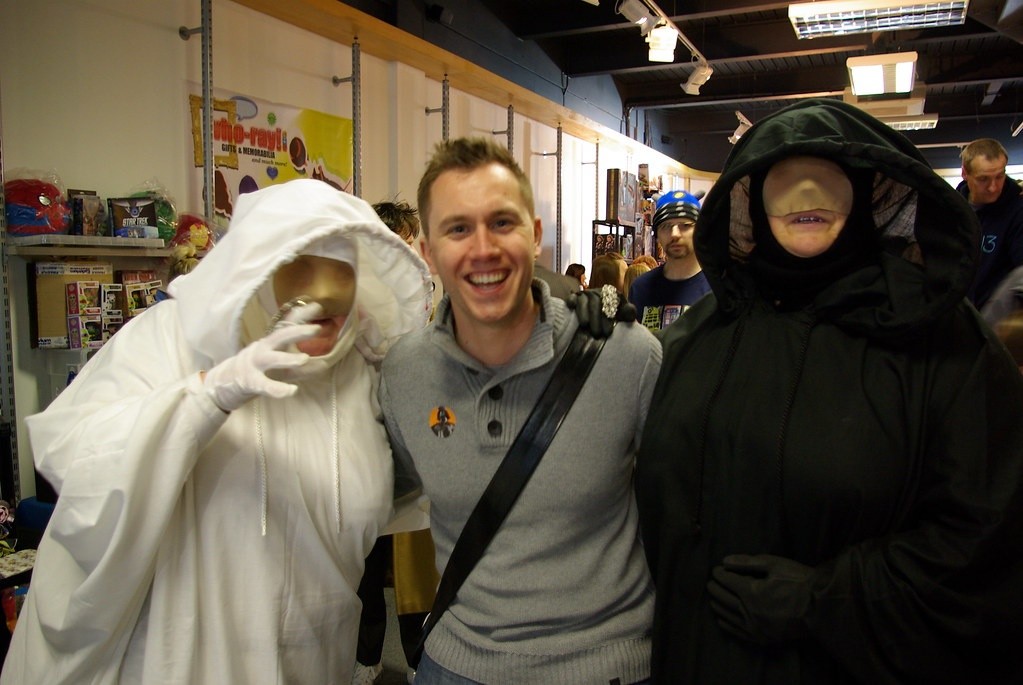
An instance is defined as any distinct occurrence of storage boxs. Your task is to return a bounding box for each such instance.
[593,162,670,267]
[26,195,170,352]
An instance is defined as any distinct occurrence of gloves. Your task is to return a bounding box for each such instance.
[204,302,324,412]
[567,284,637,340]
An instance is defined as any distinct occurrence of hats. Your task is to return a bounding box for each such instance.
[652,190,702,232]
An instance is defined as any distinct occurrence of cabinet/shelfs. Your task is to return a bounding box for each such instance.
[6,232,209,353]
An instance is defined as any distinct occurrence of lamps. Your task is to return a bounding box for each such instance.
[788,0,971,41]
[845,81,927,118]
[727,108,756,147]
[615,0,663,37]
[681,64,715,96]
[877,112,939,132]
[846,50,919,99]
[1009,116,1023,137]
[645,24,678,62]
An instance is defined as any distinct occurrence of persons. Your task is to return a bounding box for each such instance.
[356,102,1023,685]
[0,179,636,685]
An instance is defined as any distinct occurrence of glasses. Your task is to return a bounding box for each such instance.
[657,221,697,231]
